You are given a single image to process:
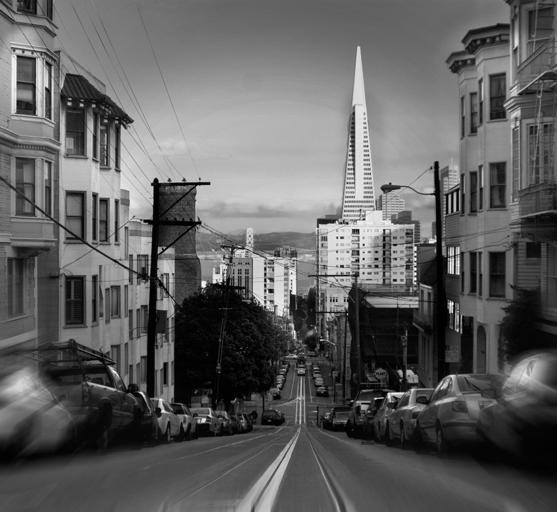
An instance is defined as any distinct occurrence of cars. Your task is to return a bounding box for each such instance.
[345,347,557,471]
[320,412,330,426]
[329,406,352,431]
[269,347,328,397]
[261,410,285,425]
[0,339,253,465]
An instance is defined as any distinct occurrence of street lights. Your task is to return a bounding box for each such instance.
[319,339,338,405]
[379,160,444,383]
